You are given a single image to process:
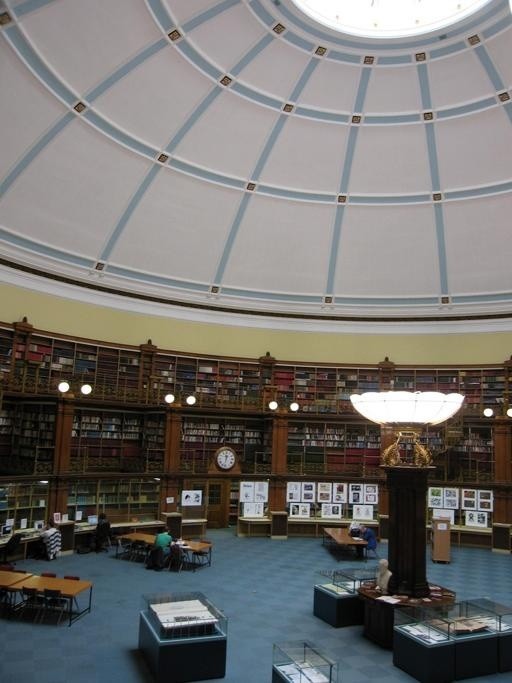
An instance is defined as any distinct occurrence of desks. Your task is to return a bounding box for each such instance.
[238,516,380,542]
[121,532,212,551]
[0,532,40,559]
[322,527,367,561]
[72,519,208,549]
[0,571,32,622]
[6,574,92,626]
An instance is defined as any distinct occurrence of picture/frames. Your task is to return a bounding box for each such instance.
[181,490,202,506]
[53,513,60,523]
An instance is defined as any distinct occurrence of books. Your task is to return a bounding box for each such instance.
[0,407,56,474]
[229,479,238,525]
[377,586,455,605]
[127,477,142,504]
[31,497,46,507]
[287,423,381,478]
[460,368,511,416]
[149,356,263,410]
[181,418,272,473]
[420,425,493,480]
[3,325,143,408]
[17,485,29,494]
[351,536,363,541]
[68,479,77,504]
[0,481,14,510]
[149,600,218,628]
[32,485,46,493]
[17,497,28,508]
[79,477,96,505]
[385,369,460,393]
[433,617,489,635]
[266,363,383,412]
[100,475,125,506]
[70,409,165,475]
[144,477,161,503]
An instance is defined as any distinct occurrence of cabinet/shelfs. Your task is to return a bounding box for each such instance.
[0,315,512,416]
[0,477,48,528]
[71,407,166,472]
[0,401,61,477]
[426,524,493,551]
[395,427,494,486]
[286,420,381,476]
[67,474,161,523]
[180,414,273,475]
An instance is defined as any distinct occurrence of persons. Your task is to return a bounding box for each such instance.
[377,559,394,593]
[40,521,61,559]
[154,527,171,558]
[359,526,378,551]
[96,513,110,531]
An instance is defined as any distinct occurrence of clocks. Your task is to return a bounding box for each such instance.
[213,446,236,471]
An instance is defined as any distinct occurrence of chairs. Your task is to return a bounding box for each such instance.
[63,576,79,612]
[16,586,48,624]
[123,539,187,574]
[0,533,26,565]
[40,572,56,577]
[365,546,379,558]
[39,588,67,627]
[191,540,210,572]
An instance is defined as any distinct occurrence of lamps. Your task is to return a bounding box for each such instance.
[349,391,465,467]
